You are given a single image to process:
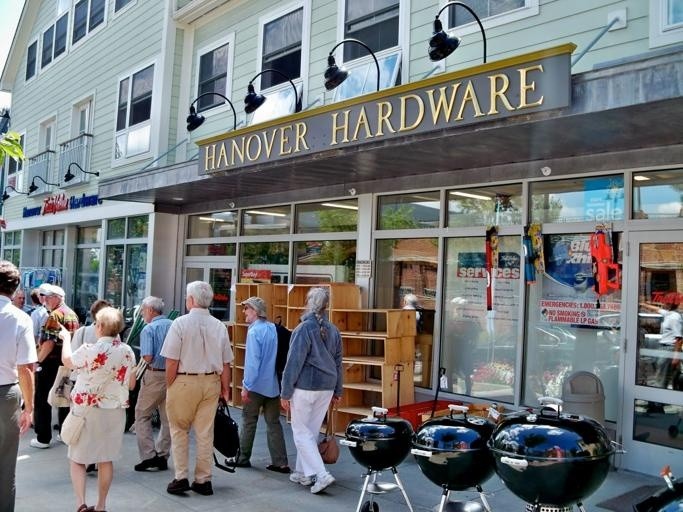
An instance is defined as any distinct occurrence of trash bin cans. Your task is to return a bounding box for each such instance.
[561,370,605,427]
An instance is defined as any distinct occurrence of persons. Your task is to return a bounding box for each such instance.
[134,295,175,471]
[12,283,52,429]
[399,291,483,397]
[30,284,81,448]
[56,307,137,512]
[636,292,683,417]
[280,286,344,494]
[0,259,39,512]
[70,299,121,473]
[158,280,235,496]
[225,296,291,475]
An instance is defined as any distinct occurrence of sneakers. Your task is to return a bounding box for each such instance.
[289,472,312,485]
[310,471,335,492]
[30,439,50,448]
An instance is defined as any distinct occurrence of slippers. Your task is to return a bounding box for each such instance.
[266,465,291,473]
[225,458,251,467]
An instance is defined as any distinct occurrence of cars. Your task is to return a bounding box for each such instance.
[590,313,664,344]
[74,271,98,309]
[475,322,616,372]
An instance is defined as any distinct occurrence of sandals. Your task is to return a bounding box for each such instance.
[77,505,88,511]
[88,506,107,512]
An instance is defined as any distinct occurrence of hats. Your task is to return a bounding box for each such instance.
[241,296,267,320]
[40,285,65,296]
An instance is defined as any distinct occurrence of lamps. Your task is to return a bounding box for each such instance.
[427,1,488,65]
[242,67,304,116]
[3,185,30,202]
[184,90,240,136]
[63,160,101,183]
[321,37,382,95]
[28,174,61,194]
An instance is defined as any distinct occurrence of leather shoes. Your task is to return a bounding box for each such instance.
[135,456,162,471]
[168,479,189,492]
[157,456,168,470]
[191,481,214,495]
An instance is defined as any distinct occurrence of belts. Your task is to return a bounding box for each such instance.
[177,371,217,375]
[147,365,165,371]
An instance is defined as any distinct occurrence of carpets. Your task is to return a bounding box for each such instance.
[595,481,664,512]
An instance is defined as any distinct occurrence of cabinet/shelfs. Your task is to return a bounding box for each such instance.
[223,274,417,439]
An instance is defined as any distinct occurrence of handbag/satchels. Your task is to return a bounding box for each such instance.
[47,366,74,408]
[318,402,339,464]
[213,397,241,473]
[61,410,87,446]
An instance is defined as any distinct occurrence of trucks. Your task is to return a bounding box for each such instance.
[248,263,348,285]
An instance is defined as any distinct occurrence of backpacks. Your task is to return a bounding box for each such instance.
[273,316,292,372]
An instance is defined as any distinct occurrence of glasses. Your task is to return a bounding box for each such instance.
[49,295,57,299]
